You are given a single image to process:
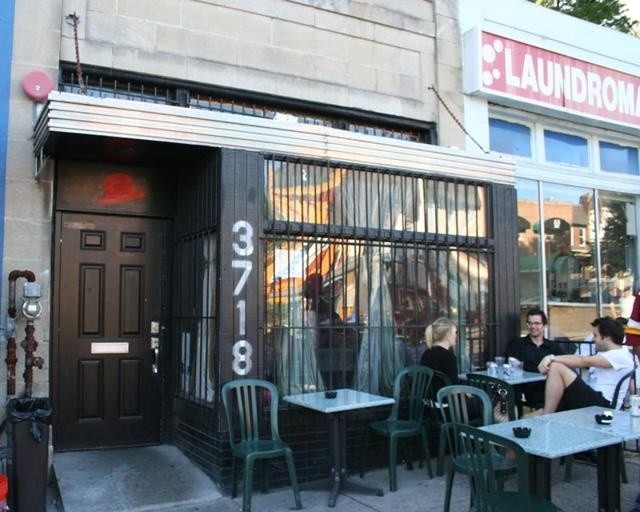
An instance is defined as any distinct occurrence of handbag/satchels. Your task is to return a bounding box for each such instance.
[487,384,519,423]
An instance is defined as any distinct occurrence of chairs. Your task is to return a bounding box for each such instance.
[436,384,517,512]
[443,423,562,512]
[418,369,482,479]
[466,374,515,422]
[360,365,434,492]
[564,372,632,484]
[221,379,303,512]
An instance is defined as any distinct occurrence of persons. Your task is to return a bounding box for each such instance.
[619,286,634,320]
[420,315,502,423]
[574,315,633,401]
[393,320,428,378]
[494,318,632,423]
[607,287,618,318]
[506,309,563,409]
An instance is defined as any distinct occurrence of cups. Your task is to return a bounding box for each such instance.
[629,395,640,417]
[485,355,525,381]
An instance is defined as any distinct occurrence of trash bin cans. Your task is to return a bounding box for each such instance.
[6,397,52,512]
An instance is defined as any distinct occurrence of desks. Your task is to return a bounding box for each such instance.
[281,389,396,508]
[539,405,640,512]
[457,367,547,420]
[477,420,622,512]
